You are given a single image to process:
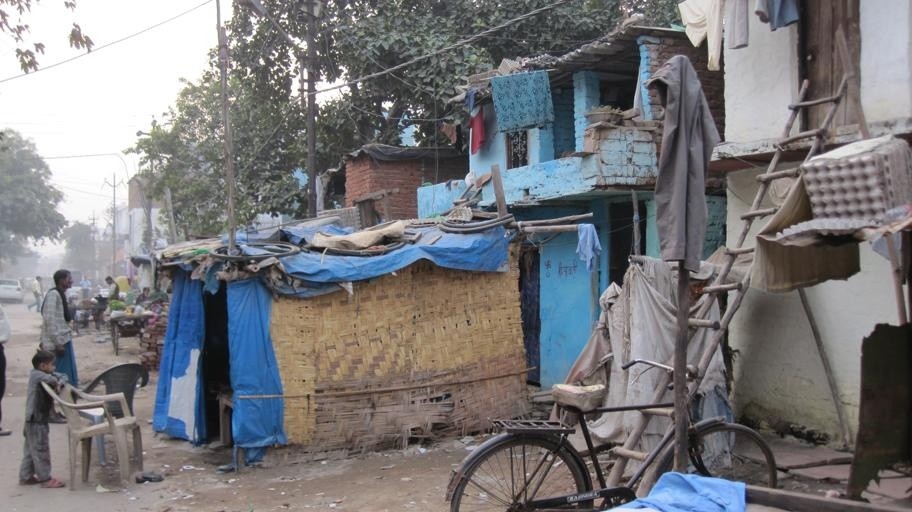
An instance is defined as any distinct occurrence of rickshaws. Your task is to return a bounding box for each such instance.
[110,310,155,356]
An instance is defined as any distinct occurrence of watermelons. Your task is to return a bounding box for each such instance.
[109,300,126,310]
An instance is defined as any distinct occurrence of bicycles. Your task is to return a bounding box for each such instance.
[444,356,778,512]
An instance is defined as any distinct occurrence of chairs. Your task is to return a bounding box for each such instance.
[41,379,143,491]
[82,364,148,479]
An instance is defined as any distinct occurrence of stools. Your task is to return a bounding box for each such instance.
[80,408,108,466]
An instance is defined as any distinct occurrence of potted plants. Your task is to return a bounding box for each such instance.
[584,105,623,125]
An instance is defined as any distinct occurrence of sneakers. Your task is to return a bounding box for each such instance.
[48,411,66,424]
[0,427,13,435]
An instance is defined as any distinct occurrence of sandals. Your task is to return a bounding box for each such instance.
[19,478,65,488]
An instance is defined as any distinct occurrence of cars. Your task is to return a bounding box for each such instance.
[0,279,22,303]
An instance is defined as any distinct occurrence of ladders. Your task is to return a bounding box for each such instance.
[599,71,848,511]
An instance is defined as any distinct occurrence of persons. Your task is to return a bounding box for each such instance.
[0,307,12,435]
[27,276,42,312]
[80,275,92,297]
[40,269,79,424]
[136,286,150,303]
[19,349,68,487]
[105,276,120,299]
